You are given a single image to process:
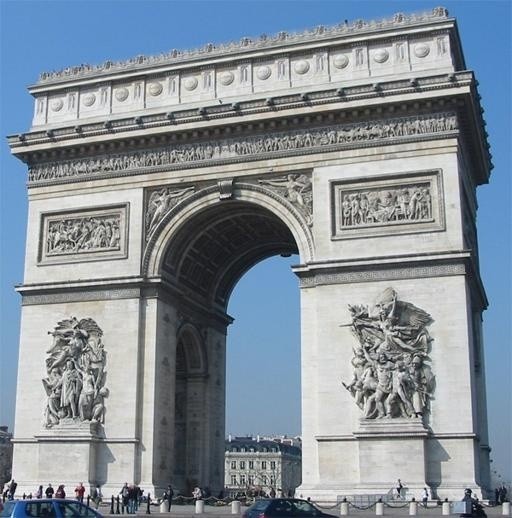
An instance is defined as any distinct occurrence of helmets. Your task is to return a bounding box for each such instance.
[464,488,473,494]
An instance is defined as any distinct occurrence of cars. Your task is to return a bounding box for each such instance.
[242,498,339,518]
[0,498,104,518]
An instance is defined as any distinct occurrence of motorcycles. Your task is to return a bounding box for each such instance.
[460,499,487,518]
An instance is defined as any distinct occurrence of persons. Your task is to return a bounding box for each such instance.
[342,188,431,226]
[36,485,42,499]
[495,485,507,505]
[192,487,202,500]
[420,488,429,510]
[472,494,479,509]
[166,485,174,512]
[40,316,110,427]
[256,172,313,226]
[7,483,17,501]
[194,488,197,506]
[339,297,432,421]
[92,485,103,510]
[55,485,65,498]
[47,218,122,255]
[45,483,54,498]
[145,184,197,243]
[118,482,143,514]
[396,479,403,498]
[74,482,84,514]
[461,488,480,518]
[270,488,276,498]
